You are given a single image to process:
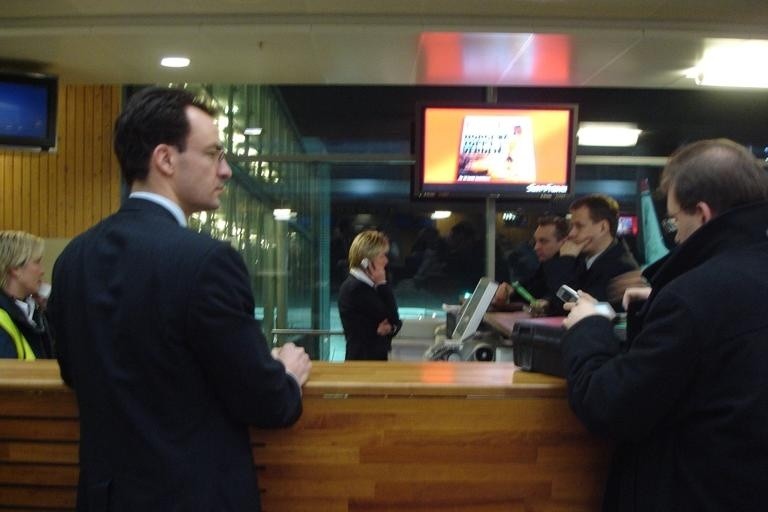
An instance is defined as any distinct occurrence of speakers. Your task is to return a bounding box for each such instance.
[447,312,455,338]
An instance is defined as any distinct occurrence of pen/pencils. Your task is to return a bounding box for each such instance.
[509,281,538,305]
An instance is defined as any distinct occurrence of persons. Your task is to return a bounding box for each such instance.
[0,228,58,361]
[335,226,403,360]
[50,83,314,511]
[554,134,767,510]
[522,191,639,318]
[486,211,569,305]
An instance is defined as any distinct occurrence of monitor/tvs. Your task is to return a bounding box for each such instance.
[616,214,639,238]
[0,69,59,153]
[410,100,580,203]
[451,277,499,343]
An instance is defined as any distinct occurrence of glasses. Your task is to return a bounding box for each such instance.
[662,217,678,231]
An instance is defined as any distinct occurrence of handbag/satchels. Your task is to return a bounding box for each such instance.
[509,313,636,377]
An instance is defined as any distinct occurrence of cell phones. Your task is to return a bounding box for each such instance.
[361,257,371,273]
[556,285,578,303]
